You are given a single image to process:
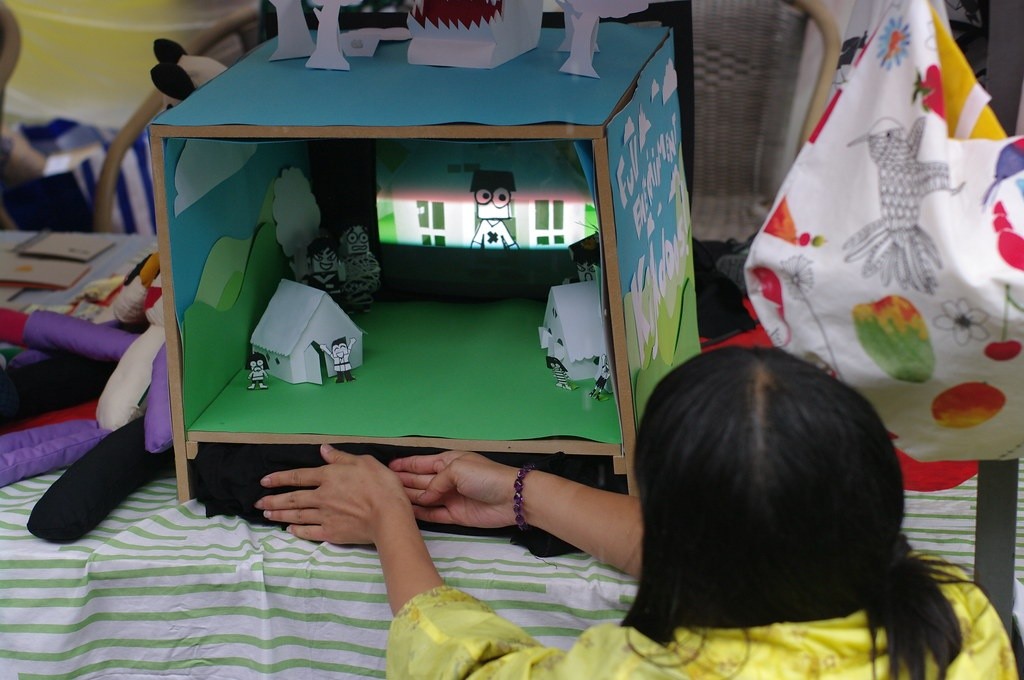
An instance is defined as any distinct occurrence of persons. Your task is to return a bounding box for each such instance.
[253,344,1022,680]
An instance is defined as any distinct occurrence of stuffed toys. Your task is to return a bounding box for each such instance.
[0,38,227,544]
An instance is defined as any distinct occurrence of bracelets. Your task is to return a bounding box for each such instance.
[513,464,536,531]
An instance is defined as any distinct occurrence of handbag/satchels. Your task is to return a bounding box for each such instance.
[743,1,1024,461]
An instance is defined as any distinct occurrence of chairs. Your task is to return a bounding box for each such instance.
[91,3,261,236]
[691,0,841,243]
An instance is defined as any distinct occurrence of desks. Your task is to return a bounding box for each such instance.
[0,230,1024,680]
